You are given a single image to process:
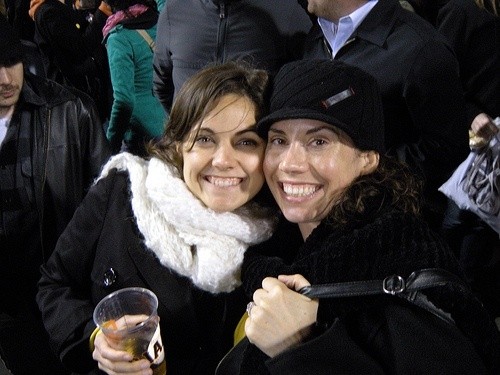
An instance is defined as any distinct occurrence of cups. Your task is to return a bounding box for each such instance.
[93,286,166,375]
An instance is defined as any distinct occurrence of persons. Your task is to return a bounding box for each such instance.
[153,1,314,110]
[305,0,476,197]
[435,1,500,118]
[214,59,500,375]
[102,1,171,158]
[0,11,112,374]
[0,0,112,115]
[37,60,284,375]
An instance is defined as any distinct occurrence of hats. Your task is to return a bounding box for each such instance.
[0,12,36,70]
[256,57,383,151]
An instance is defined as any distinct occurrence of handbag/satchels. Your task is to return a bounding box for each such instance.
[436,117,500,238]
